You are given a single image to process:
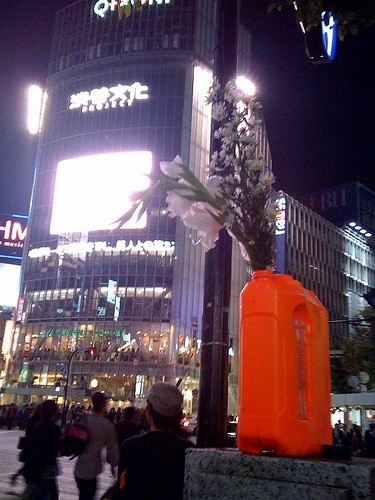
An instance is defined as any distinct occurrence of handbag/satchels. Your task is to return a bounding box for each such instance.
[60,426,91,461]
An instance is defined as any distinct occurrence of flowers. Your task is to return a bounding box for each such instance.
[111,76,285,270]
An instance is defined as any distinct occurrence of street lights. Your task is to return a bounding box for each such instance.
[54,347,95,429]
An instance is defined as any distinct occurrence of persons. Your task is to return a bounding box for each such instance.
[0,346,239,489]
[71,392,119,500]
[18,400,63,500]
[331,418,375,458]
[99,383,197,500]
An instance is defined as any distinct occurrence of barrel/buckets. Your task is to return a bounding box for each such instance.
[236,269,336,458]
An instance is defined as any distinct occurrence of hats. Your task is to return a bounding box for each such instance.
[147,382,184,419]
